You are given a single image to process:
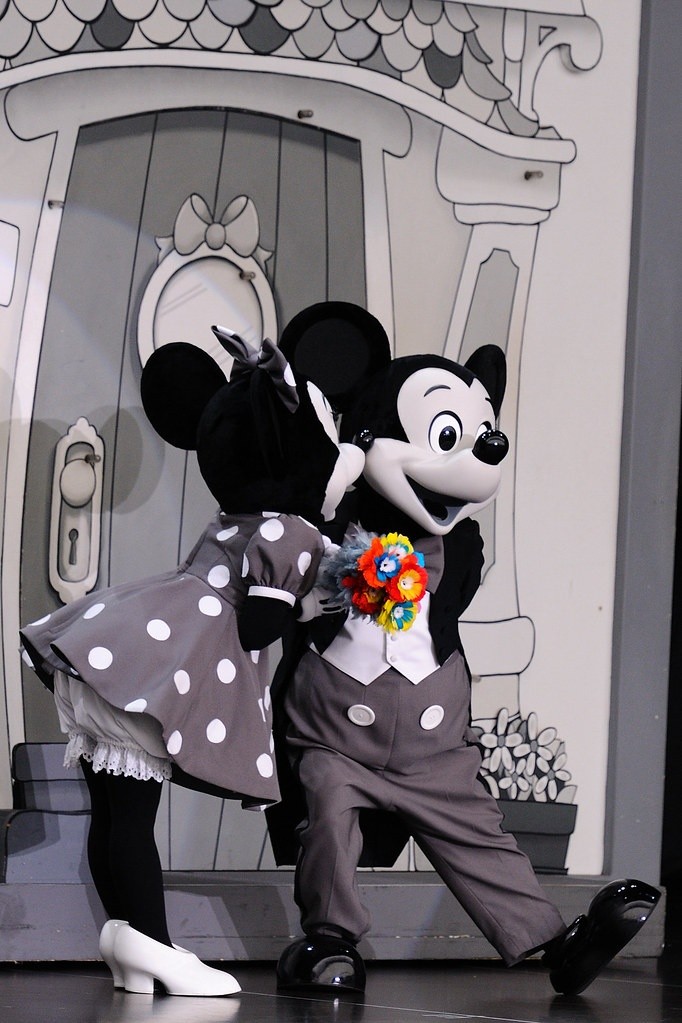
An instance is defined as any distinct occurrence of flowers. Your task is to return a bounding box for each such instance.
[326,520,429,633]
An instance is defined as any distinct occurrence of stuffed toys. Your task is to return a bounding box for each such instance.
[17,325,374,996]
[265,299,661,999]
